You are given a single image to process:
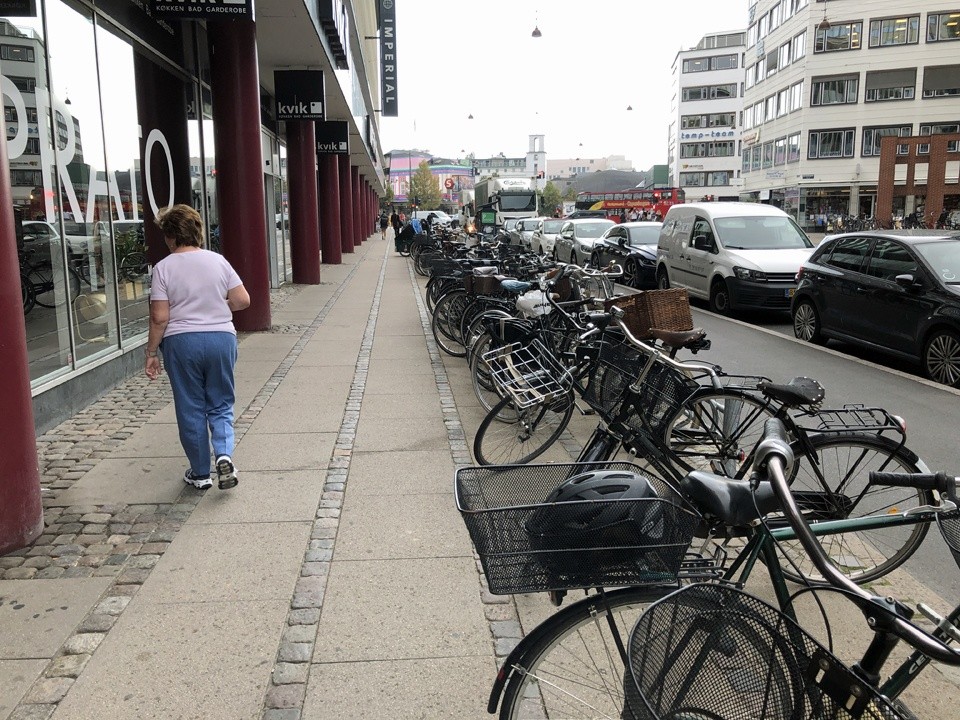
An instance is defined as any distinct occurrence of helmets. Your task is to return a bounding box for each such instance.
[523,469,663,576]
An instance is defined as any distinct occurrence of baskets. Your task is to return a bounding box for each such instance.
[412,224,615,409]
[602,287,694,340]
[581,332,701,443]
[620,583,910,720]
[454,460,702,595]
[935,509,960,569]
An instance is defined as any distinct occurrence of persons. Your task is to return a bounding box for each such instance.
[380,210,405,240]
[620,207,655,224]
[145,204,251,489]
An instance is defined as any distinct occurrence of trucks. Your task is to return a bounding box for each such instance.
[458,190,474,226]
[474,177,538,229]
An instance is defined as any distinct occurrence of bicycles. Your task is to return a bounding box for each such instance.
[825,211,960,235]
[400,221,960,720]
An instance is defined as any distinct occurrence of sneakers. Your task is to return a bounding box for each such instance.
[215,454,238,490]
[183,469,214,490]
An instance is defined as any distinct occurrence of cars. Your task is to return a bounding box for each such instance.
[499,219,522,243]
[510,218,553,254]
[552,218,618,266]
[531,217,575,260]
[790,230,960,387]
[589,222,664,290]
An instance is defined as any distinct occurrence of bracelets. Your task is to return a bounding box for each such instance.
[145,349,158,357]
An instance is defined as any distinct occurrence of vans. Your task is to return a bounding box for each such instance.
[654,201,817,320]
[561,210,608,219]
[412,211,452,223]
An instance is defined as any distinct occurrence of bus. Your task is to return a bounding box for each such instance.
[575,187,686,224]
